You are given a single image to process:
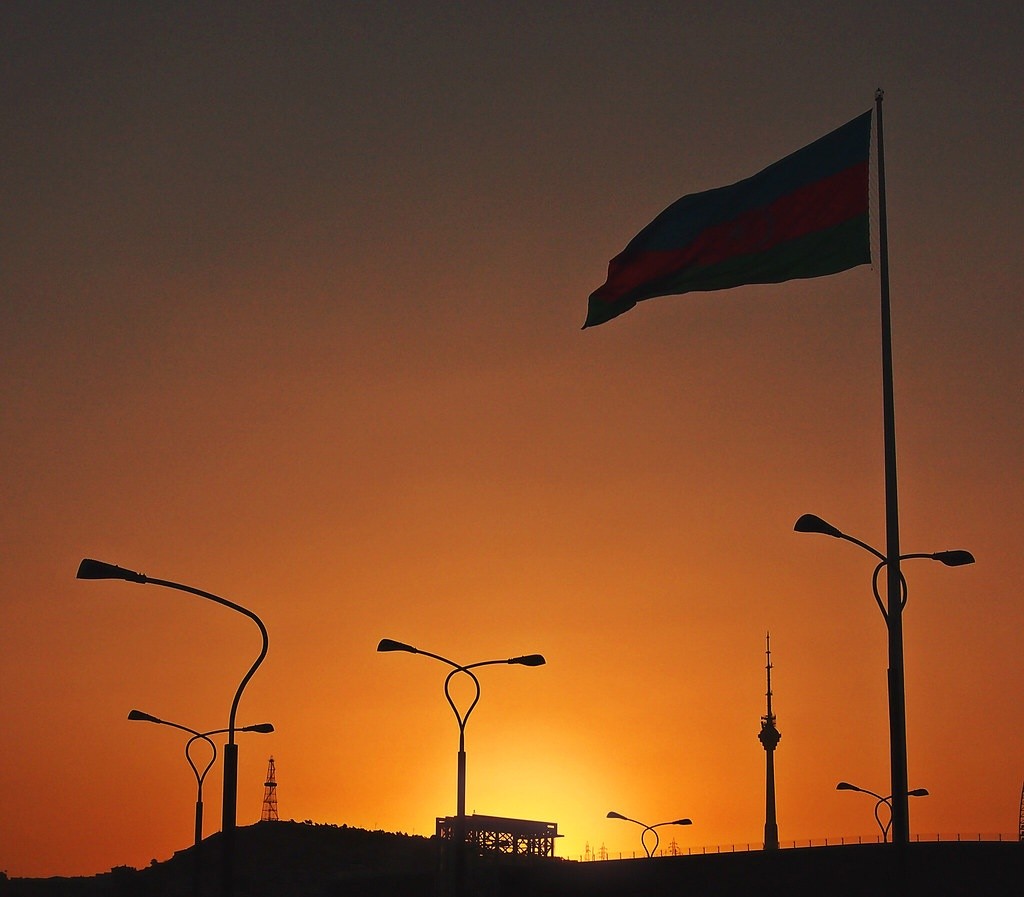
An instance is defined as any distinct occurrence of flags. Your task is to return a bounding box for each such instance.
[581,107,874,334]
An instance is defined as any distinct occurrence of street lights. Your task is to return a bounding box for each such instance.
[128,710,276,896]
[377,636,548,897]
[833,779,930,846]
[77,557,270,896]
[790,512,976,873]
[605,811,693,861]
